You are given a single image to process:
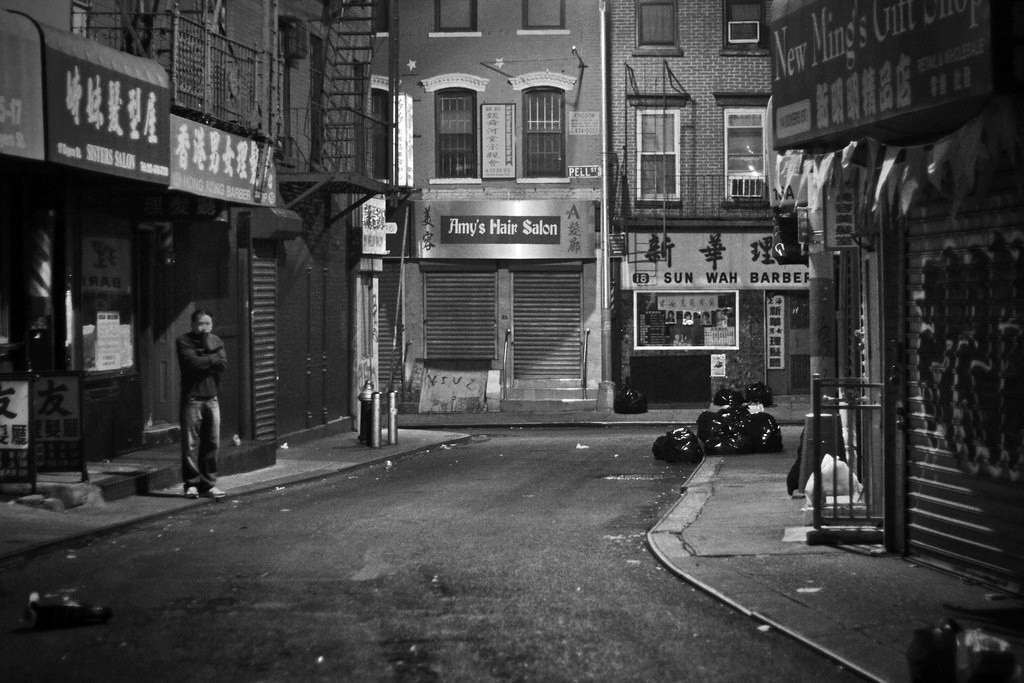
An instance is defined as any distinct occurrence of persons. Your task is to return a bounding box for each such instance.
[176,309,227,499]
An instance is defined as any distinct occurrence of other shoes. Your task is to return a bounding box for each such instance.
[186,486,199,498]
[205,486,226,497]
